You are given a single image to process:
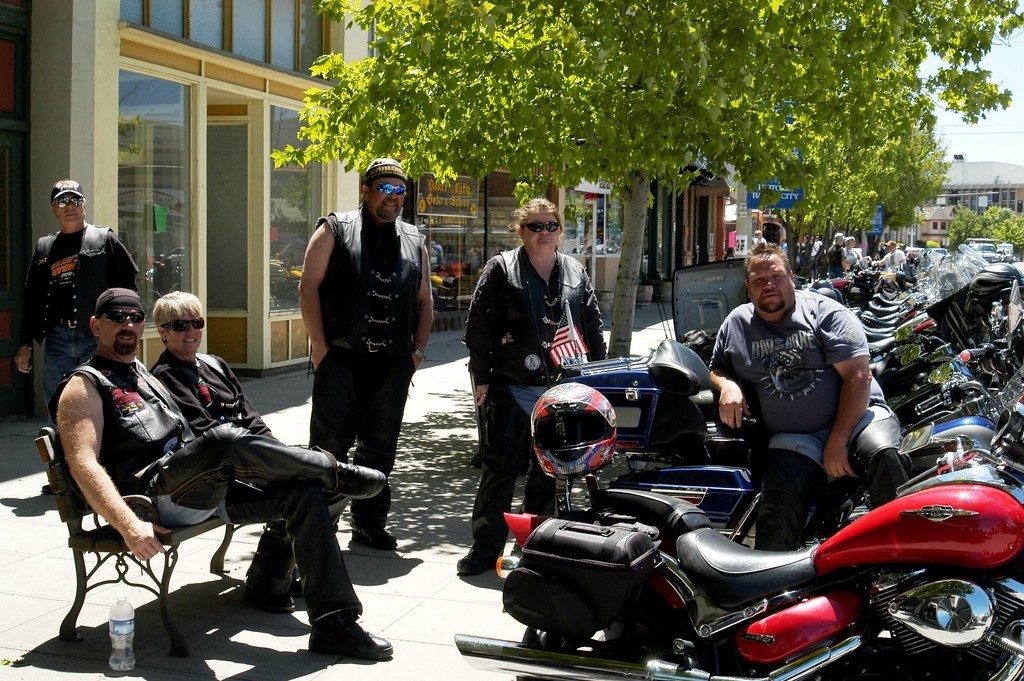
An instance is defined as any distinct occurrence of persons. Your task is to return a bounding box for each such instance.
[56,289,395,659]
[710,244,911,553]
[725,230,913,280]
[14,183,142,492]
[456,198,607,574]
[428,238,514,276]
[147,293,347,613]
[298,158,435,551]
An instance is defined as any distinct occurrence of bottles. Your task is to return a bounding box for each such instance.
[108,595,136,671]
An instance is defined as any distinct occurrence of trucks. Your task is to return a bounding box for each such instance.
[996,244,1014,264]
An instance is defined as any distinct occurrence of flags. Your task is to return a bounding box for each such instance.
[549,301,590,366]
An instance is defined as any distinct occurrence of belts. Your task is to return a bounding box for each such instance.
[50,319,83,329]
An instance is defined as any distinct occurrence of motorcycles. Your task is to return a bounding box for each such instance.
[454,244,1024,681]
[135,250,181,301]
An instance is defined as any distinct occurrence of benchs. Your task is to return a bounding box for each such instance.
[34,424,250,660]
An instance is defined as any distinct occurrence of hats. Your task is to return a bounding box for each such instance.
[363,159,408,185]
[51,181,86,203]
[94,288,144,318]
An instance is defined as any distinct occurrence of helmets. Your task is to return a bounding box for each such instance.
[533,384,619,475]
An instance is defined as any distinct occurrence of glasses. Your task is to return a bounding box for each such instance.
[104,309,146,323]
[521,221,561,233]
[54,198,84,209]
[159,317,205,331]
[371,184,406,194]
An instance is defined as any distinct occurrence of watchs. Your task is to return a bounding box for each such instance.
[411,351,427,362]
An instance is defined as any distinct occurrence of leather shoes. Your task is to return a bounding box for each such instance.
[244,586,296,614]
[335,461,387,499]
[286,579,303,597]
[352,523,397,551]
[309,619,392,660]
[458,550,499,574]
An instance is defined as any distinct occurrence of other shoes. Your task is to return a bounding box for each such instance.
[41,485,57,495]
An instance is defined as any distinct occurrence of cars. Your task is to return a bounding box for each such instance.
[969,244,1001,263]
[270,261,302,281]
[160,247,184,260]
[923,248,948,254]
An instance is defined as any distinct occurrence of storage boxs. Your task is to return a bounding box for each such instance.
[555,357,660,442]
[671,257,747,343]
[504,514,656,636]
